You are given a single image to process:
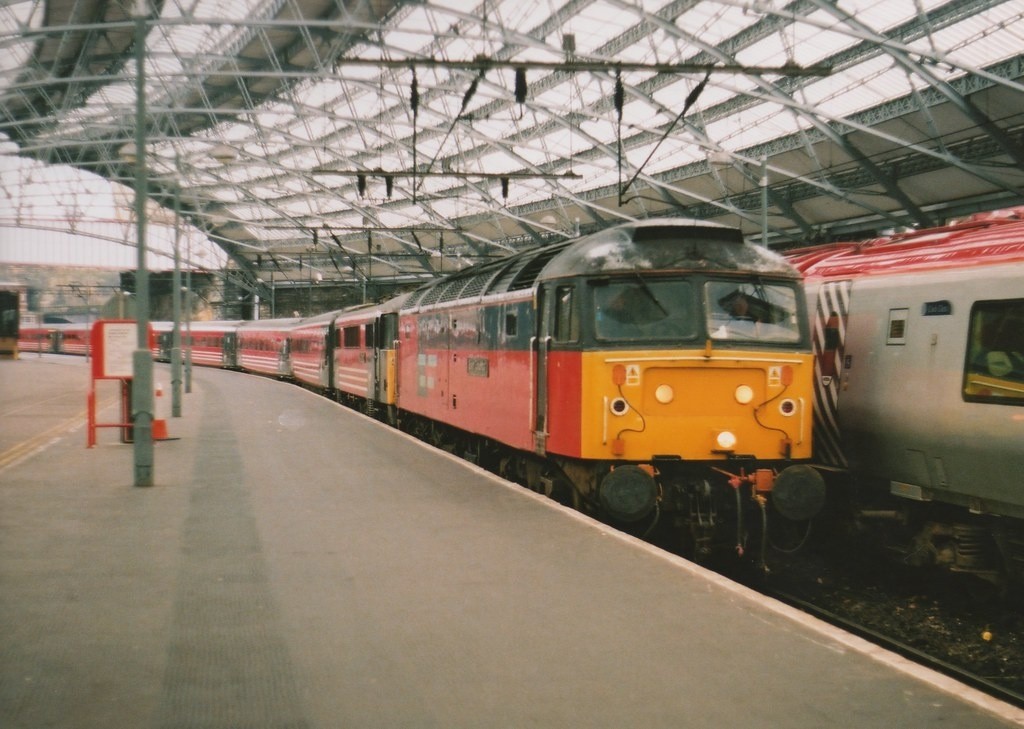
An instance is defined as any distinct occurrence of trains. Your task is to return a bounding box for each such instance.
[19,204,1024,623]
